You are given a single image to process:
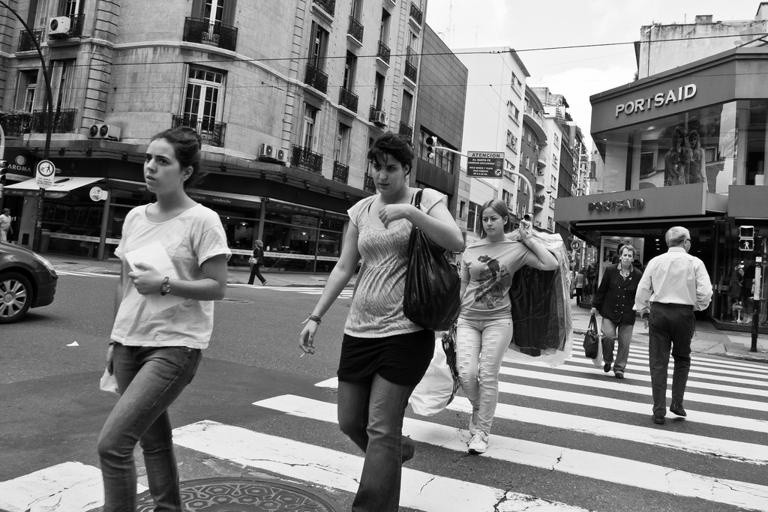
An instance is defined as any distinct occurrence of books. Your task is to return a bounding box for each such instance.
[124,241,181,284]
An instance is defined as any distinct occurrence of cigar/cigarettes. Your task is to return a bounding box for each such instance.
[299,352,305,359]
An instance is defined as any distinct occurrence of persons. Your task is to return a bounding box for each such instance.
[247,239,269,286]
[589,243,643,380]
[296,130,466,512]
[92,122,235,512]
[663,125,690,186]
[573,266,586,307]
[0,207,14,242]
[454,196,560,455]
[686,129,710,193]
[631,224,714,425]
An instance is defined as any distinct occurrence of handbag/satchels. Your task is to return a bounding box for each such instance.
[402,227,461,332]
[583,329,599,359]
[249,257,257,264]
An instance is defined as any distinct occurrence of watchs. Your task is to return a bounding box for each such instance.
[160,275,172,296]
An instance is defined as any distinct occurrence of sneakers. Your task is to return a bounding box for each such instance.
[469,408,490,456]
[263,281,267,286]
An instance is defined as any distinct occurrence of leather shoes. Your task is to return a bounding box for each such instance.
[670,401,686,416]
[604,363,611,372]
[615,373,623,379]
[652,414,665,425]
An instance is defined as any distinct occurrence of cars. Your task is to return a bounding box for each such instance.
[0,241,58,325]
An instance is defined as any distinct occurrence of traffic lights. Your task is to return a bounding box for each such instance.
[738,225,755,252]
[0,160,7,185]
[424,136,436,160]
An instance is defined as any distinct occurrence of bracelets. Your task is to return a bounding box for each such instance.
[310,314,321,324]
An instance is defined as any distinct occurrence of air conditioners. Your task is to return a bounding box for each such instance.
[275,147,289,162]
[259,143,277,160]
[375,111,387,126]
[48,15,71,35]
[87,124,120,141]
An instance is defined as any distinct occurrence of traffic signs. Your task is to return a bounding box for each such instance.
[467,150,504,179]
